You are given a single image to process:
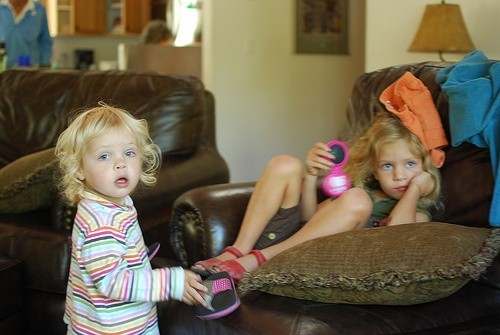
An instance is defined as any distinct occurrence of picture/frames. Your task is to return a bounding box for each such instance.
[292,0,351,56]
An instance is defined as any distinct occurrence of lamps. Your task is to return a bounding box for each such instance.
[408,0,477,62]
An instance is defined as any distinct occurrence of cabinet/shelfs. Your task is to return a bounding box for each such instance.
[45,0,173,38]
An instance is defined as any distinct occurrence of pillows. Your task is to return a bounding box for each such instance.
[239,220,500,307]
[0,143,59,218]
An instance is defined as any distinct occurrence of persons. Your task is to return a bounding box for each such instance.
[141,20,174,46]
[0,0,53,68]
[110,16,121,33]
[191,116,441,285]
[54,104,208,335]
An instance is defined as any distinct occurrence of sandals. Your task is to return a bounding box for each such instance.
[204,250,269,285]
[188,245,246,279]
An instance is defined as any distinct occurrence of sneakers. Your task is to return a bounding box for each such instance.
[184,268,239,319]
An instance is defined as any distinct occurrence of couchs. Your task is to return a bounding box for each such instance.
[168,61,500,335]
[0,63,233,335]
